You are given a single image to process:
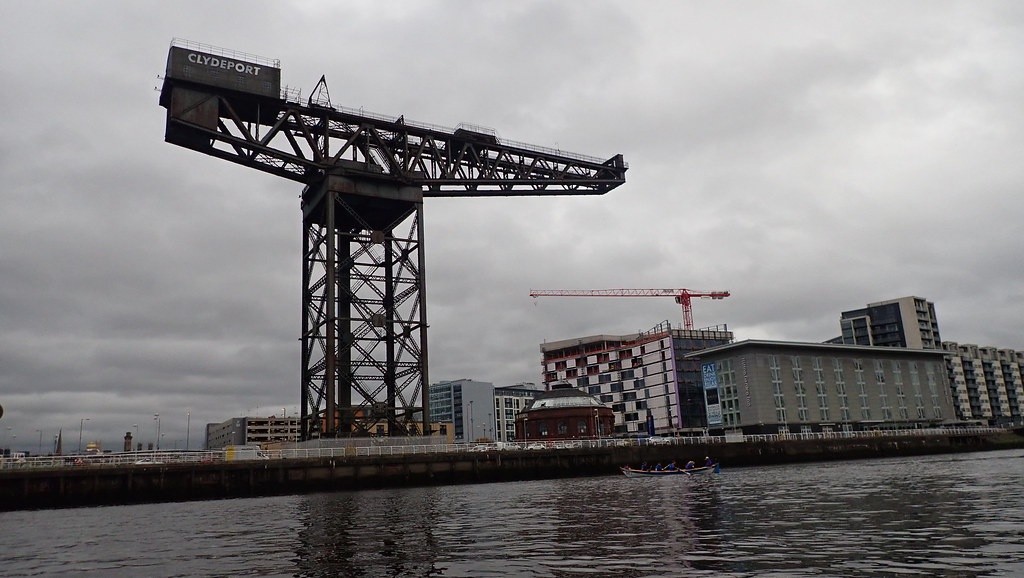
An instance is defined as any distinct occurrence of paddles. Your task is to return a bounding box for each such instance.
[678,468,690,476]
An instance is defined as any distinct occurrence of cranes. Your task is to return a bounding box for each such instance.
[529,288,730,330]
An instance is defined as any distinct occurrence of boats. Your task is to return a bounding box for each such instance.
[619,461,719,477]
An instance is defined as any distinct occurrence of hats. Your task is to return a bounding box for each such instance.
[705,457,709,460]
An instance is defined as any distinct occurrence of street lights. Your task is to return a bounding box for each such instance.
[594,408,601,439]
[524,418,528,448]
[79,418,90,452]
[36,429,42,455]
[153,412,160,448]
[132,423,139,461]
[186,411,190,450]
[467,400,474,443]
